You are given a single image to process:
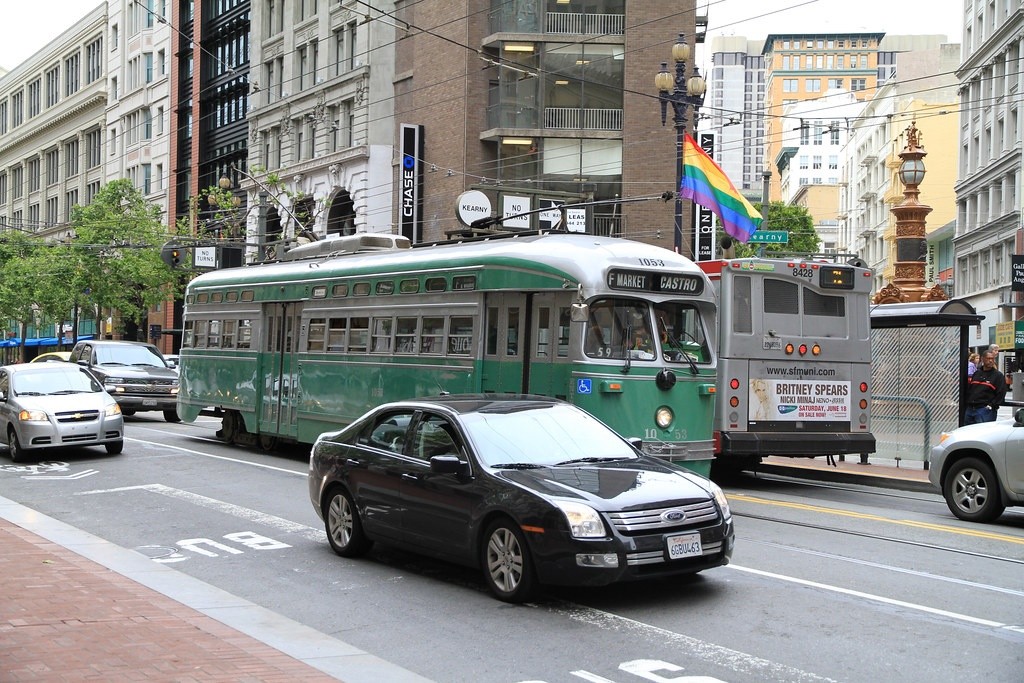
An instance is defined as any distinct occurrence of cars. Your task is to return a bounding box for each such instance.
[0,362,124,463]
[306,392,736,603]
[160,354,180,372]
[29,352,71,363]
[928,408,1023,523]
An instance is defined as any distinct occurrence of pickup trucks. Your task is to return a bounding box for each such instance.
[47,340,179,423]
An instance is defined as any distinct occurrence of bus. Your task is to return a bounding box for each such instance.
[682,258,876,487]
[175,233,718,484]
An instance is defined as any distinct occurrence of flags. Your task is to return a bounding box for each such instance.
[680,130,763,245]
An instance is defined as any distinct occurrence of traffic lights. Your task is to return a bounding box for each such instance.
[171,246,180,266]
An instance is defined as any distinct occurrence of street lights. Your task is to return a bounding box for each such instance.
[655,33,707,343]
[208,173,241,269]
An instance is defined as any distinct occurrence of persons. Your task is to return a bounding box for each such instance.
[590,327,605,353]
[964,345,1006,426]
[628,311,668,351]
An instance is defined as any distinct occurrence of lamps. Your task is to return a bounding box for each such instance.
[504,42,534,51]
[502,137,534,145]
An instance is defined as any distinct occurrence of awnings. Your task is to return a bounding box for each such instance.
[0,335,93,347]
